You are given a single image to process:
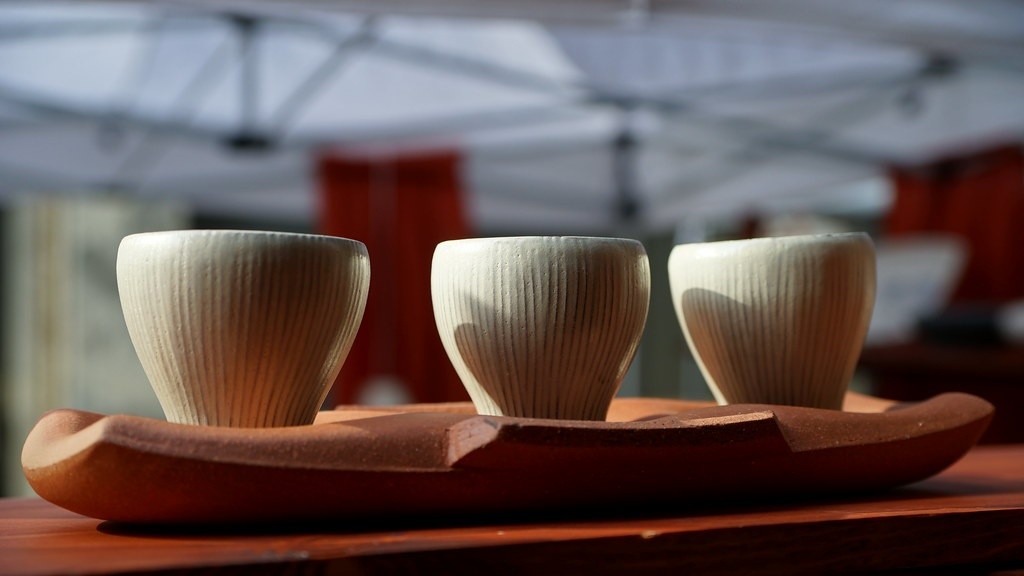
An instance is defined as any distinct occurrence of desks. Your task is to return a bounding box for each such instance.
[0,446,1024,576]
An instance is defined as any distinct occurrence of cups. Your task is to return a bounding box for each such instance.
[117,232,371,426]
[431,237,650,421]
[667,235,876,411]
